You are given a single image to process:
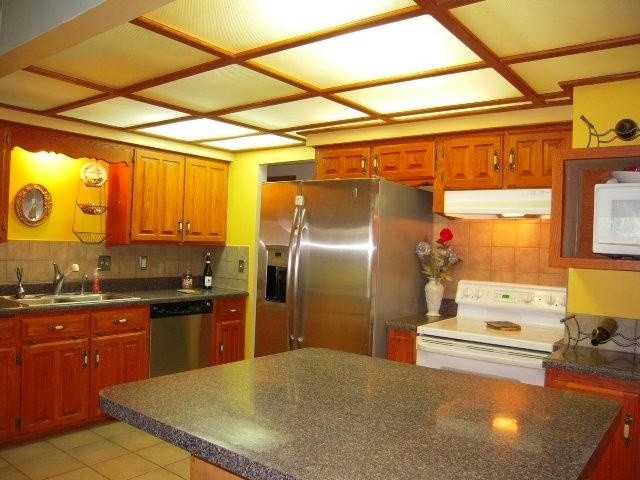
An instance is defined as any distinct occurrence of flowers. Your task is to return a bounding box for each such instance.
[411,227,459,284]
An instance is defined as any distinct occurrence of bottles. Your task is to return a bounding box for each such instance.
[181,270,194,289]
[591,318,618,346]
[616,119,636,137]
[203,252,213,289]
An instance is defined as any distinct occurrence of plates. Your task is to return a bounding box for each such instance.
[610,170,640,182]
[80,163,108,187]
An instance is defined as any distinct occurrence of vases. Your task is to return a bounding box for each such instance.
[422,275,447,319]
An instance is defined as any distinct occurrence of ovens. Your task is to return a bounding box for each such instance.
[416,334,545,386]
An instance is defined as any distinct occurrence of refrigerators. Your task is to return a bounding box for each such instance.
[252,179,435,362]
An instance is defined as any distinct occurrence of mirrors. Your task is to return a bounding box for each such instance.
[14,184,53,227]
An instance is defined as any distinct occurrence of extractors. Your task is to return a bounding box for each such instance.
[444,187,551,219]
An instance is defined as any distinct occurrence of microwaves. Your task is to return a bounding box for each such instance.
[591,183,640,259]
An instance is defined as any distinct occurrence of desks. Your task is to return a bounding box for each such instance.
[100,346,623,479]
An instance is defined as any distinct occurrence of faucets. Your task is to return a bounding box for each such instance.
[81,273,88,295]
[51,262,80,295]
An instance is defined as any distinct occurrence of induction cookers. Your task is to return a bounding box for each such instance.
[417,313,567,355]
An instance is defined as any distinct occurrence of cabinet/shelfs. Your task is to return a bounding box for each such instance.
[436,122,572,192]
[308,133,444,190]
[550,147,639,270]
[211,290,249,364]
[19,302,158,447]
[104,144,233,248]
[1,311,24,451]
[387,331,419,362]
[545,366,639,480]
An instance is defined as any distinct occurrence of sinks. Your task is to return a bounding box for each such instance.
[0,293,81,306]
[70,292,141,305]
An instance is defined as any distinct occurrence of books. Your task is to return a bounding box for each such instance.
[486,319,521,333]
